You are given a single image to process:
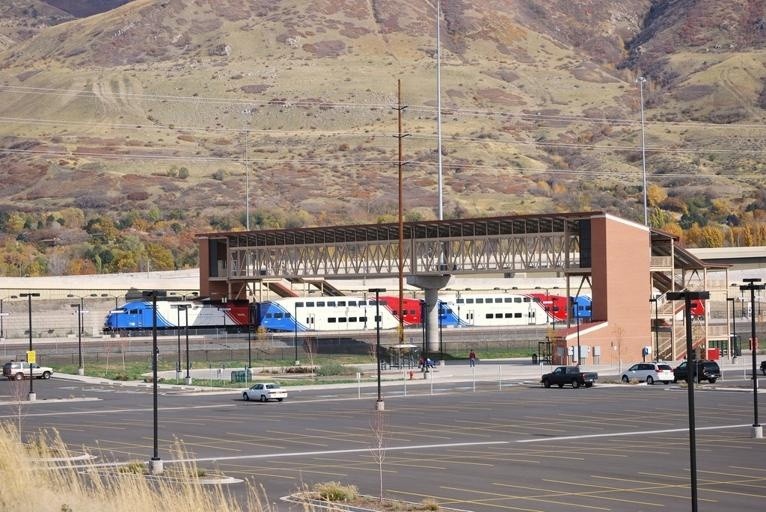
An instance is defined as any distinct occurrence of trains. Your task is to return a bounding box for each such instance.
[244,288,591,334]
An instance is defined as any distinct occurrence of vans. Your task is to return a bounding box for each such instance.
[621,360,675,387]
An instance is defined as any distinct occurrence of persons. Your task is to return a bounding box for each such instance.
[469,348,478,367]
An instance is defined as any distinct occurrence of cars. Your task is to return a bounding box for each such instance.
[759,358,765,375]
[242,383,288,401]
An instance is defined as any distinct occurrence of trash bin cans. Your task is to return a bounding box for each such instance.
[532,354,537,364]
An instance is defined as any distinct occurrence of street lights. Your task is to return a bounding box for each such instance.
[738,277,765,440]
[19,292,42,401]
[138,287,171,476]
[667,287,712,510]
[240,108,255,229]
[369,288,387,411]
[1,295,19,342]
[67,294,99,337]
[247,304,260,381]
[71,304,86,375]
[172,304,195,384]
[635,76,649,227]
[101,293,130,338]
[726,297,738,364]
[293,302,301,366]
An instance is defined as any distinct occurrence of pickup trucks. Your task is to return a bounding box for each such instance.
[539,365,598,390]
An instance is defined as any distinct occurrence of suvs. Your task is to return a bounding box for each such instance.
[2,361,55,381]
[672,358,723,386]
